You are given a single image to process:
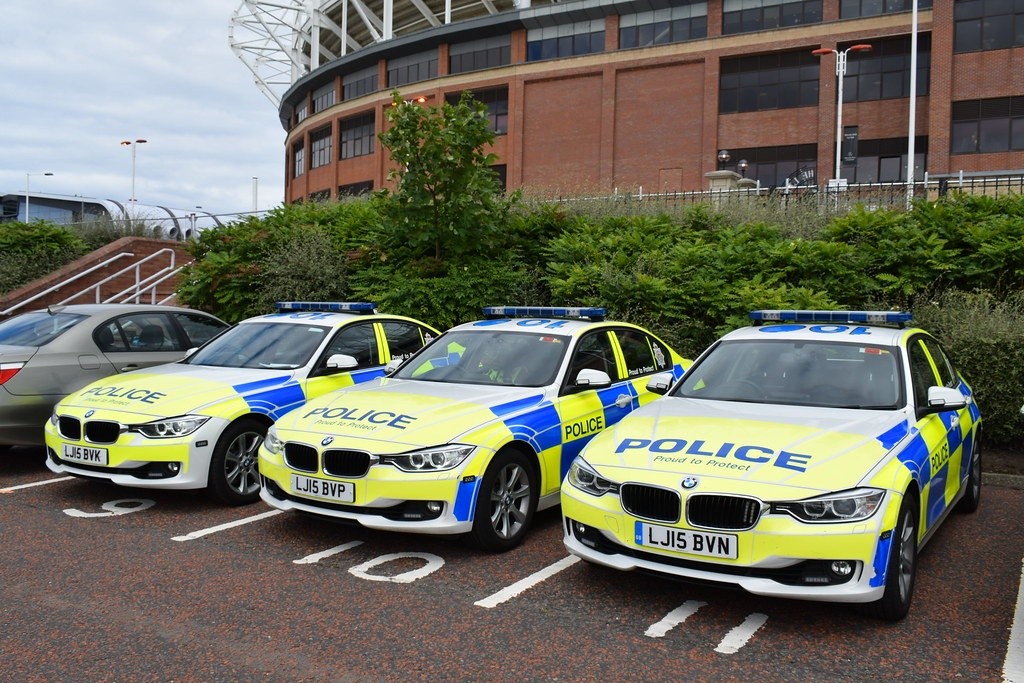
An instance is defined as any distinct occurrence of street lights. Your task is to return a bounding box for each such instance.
[392,95,428,173]
[25,173,54,224]
[121,139,148,232]
[811,44,872,179]
[252,177,258,211]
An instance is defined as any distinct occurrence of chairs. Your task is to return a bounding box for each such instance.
[96,327,119,350]
[564,349,608,387]
[124,327,137,351]
[136,325,164,351]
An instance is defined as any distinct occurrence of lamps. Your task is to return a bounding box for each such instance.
[391,101,397,107]
[417,96,427,103]
[718,150,730,170]
[737,160,748,178]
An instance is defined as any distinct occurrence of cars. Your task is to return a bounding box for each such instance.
[560,309,984,622]
[44,302,443,507]
[258,307,705,555]
[0,303,231,452]
